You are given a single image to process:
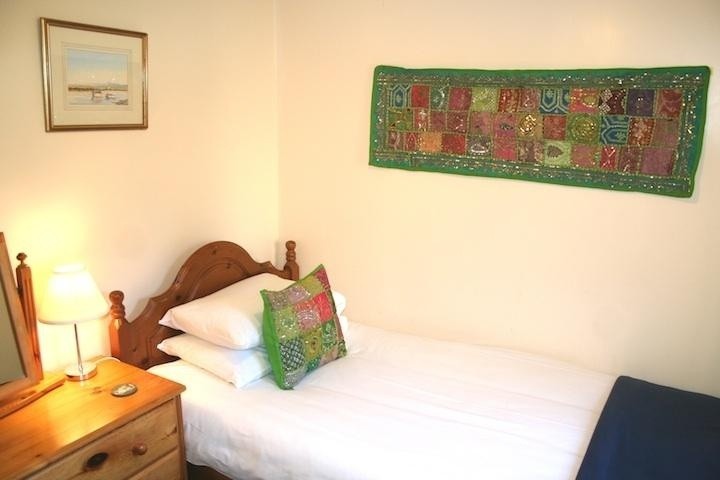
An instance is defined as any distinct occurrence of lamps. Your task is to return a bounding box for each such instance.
[33,264,112,382]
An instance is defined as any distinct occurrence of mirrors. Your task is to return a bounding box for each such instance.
[0,229,41,404]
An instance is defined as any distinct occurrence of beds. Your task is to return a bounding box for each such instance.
[108,238,718,478]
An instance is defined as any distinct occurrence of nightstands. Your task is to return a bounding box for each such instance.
[1,354,189,480]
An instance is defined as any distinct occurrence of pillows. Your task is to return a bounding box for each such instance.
[154,262,350,390]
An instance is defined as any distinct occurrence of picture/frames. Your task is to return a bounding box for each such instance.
[38,16,148,133]
